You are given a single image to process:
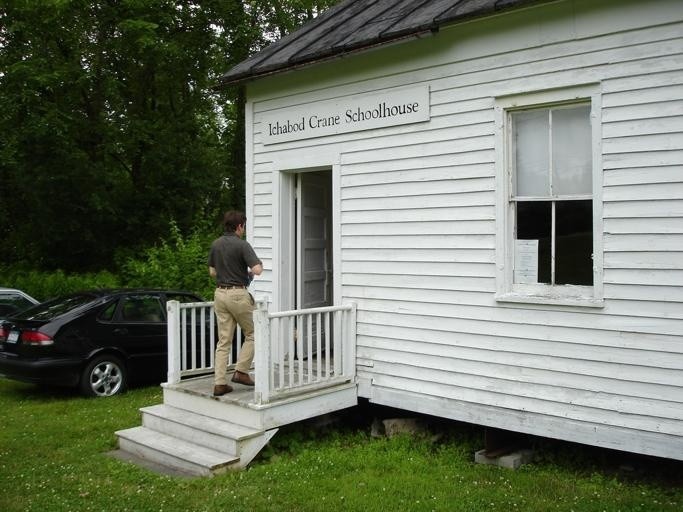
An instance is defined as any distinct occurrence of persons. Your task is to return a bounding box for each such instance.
[208,210,264,397]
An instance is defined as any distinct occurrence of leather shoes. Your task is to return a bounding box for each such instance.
[214,384,233,396]
[231,370,254,386]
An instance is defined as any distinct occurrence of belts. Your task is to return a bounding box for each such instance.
[217,285,247,289]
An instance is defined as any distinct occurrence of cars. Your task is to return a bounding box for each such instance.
[0,287,244,398]
